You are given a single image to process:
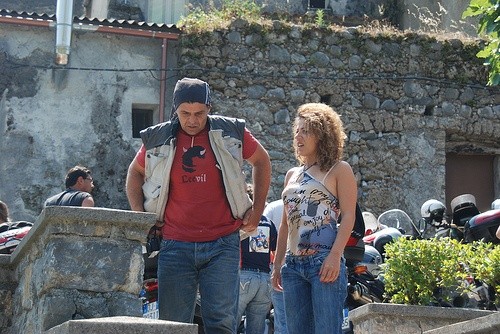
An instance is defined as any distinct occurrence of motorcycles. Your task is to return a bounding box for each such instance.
[348,194,500,309]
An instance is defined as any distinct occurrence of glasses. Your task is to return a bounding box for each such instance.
[85,178,94,184]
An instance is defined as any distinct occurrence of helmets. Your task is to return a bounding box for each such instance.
[421,199,445,219]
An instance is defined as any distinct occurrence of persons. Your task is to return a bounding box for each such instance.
[46,165,95,207]
[126,77,358,334]
[0,201,9,223]
